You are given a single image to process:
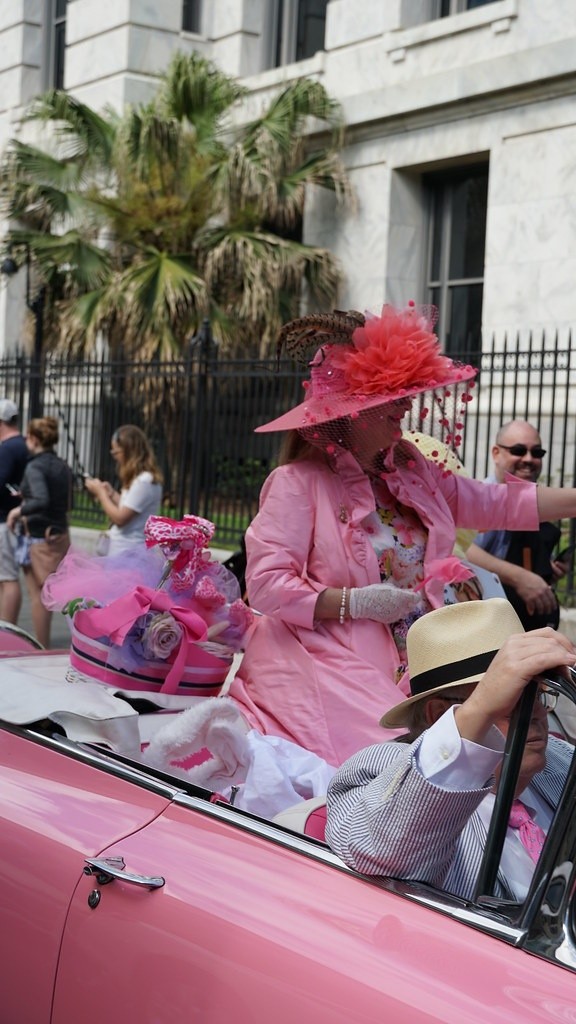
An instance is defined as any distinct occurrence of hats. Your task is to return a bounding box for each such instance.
[0,399,18,421]
[378,598,527,730]
[254,298,479,496]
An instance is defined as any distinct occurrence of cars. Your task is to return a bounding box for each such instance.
[0,624,576,1024]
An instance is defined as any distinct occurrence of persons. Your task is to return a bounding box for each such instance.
[231,300,576,767]
[6,416,71,649]
[85,425,164,556]
[0,399,30,627]
[324,597,576,901]
[466,421,570,632]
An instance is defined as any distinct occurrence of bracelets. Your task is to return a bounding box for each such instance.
[110,490,114,499]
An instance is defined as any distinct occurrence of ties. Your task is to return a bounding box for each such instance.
[508,800,547,864]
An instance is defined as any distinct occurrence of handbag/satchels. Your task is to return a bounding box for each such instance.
[30,525,70,587]
[95,534,110,556]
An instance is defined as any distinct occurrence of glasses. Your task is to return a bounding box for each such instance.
[499,445,546,458]
[437,688,560,720]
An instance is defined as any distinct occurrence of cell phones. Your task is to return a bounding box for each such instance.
[6,483,20,494]
[82,472,94,480]
[551,543,576,573]
